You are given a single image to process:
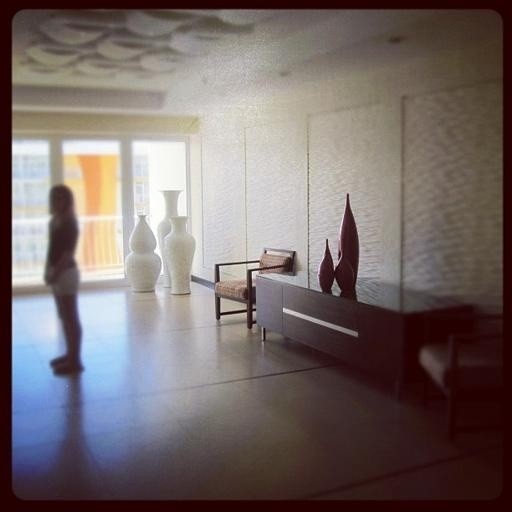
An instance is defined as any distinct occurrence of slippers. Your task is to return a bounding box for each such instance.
[49,355,84,375]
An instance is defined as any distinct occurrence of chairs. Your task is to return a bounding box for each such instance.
[418,313,504,431]
[214,247,297,329]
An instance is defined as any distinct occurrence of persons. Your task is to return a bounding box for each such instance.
[45,184,85,375]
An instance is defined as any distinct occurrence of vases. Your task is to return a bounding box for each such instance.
[124,190,196,295]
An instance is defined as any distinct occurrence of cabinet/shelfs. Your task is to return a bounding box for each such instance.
[256,276,473,404]
